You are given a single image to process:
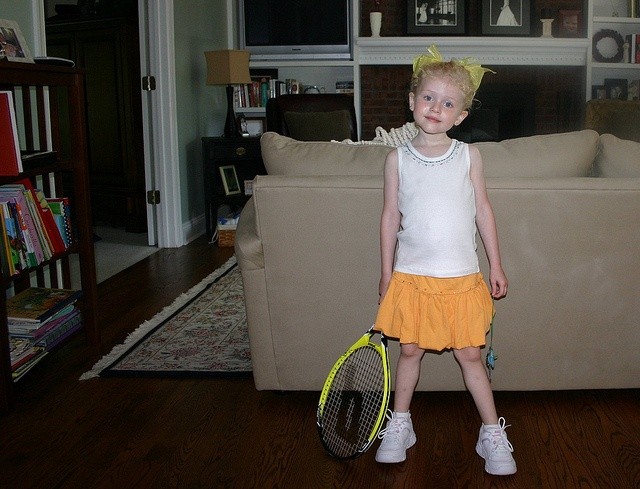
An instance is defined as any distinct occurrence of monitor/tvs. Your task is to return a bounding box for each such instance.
[235,0,352,61]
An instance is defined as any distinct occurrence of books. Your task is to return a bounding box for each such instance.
[0,90,24,176]
[0,179,73,275]
[234,78,301,109]
[6,286,84,382]
[627,34,639,63]
[629,0,636,17]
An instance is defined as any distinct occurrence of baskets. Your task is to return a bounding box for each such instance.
[218,223,236,248]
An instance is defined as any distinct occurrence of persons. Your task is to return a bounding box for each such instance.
[495,0,521,26]
[375,44,518,476]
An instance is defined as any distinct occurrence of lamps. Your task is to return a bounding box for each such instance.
[200,49,254,140]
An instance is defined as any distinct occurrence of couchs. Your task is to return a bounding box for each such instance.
[230,129,639,396]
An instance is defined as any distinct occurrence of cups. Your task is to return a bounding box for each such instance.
[369,12,382,38]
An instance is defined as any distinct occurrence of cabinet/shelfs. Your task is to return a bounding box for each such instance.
[44,9,148,235]
[0,55,103,396]
[233,59,361,142]
[586,2,638,140]
[199,132,268,242]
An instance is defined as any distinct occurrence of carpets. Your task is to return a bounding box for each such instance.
[77,249,254,386]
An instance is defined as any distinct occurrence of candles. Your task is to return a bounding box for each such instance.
[540,7,553,20]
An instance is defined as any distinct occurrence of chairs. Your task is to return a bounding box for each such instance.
[264,92,360,145]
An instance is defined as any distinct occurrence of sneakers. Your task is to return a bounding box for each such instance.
[375,409,417,463]
[475,417,517,475]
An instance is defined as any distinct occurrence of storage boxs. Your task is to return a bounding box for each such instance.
[215,217,241,246]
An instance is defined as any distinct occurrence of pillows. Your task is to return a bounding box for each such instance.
[591,133,638,181]
[447,126,600,180]
[258,129,397,181]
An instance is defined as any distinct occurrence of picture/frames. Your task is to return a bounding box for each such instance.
[603,77,631,103]
[0,18,34,64]
[476,0,537,37]
[404,0,472,38]
[557,9,584,38]
[590,85,613,101]
